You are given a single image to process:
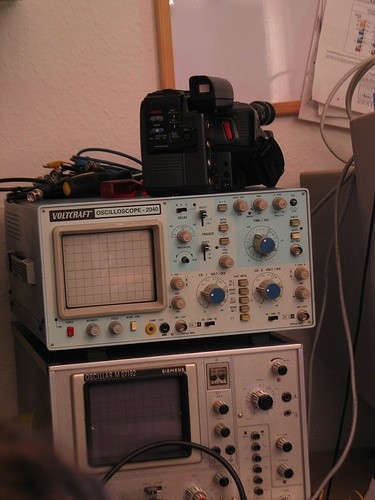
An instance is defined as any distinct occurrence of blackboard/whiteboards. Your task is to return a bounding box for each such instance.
[152,0,320,117]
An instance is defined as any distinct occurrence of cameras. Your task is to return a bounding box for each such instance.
[140,76,287,195]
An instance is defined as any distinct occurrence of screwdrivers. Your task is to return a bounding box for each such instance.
[63,166,142,199]
